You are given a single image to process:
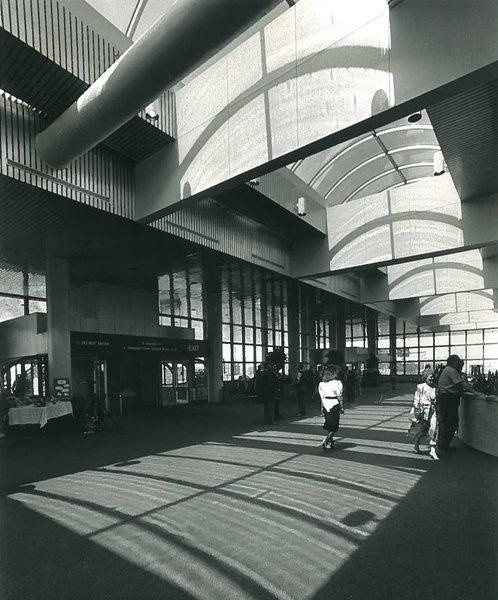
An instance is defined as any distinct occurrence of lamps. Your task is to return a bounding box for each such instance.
[296,196,307,217]
[432,151,448,177]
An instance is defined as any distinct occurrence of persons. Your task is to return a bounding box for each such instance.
[316,364,344,450]
[436,353,483,452]
[12,374,21,391]
[407,370,440,461]
[235,355,370,427]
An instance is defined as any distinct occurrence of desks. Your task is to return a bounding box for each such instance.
[6,400,75,437]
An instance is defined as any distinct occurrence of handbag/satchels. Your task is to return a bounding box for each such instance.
[409,404,433,421]
